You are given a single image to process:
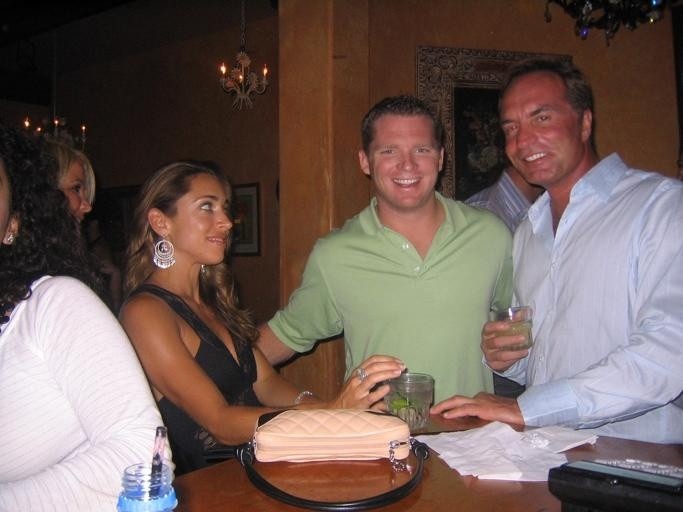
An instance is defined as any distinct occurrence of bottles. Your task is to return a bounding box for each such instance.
[117,463,178,511]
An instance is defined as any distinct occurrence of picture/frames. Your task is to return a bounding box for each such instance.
[231,182,260,257]
[416,46,573,202]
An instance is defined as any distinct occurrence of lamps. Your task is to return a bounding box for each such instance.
[22,53,98,164]
[215,0,270,111]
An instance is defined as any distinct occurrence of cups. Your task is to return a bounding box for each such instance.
[384,374,435,430]
[489,299,532,351]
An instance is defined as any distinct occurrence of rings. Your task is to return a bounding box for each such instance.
[356,368,368,380]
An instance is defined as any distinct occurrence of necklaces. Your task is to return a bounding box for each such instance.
[295,391,313,406]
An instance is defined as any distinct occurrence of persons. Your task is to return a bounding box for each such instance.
[54,141,97,223]
[430,59,683,446]
[254,93,513,409]
[463,162,545,399]
[0,125,173,512]
[114,160,407,476]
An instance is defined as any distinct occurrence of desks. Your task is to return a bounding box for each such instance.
[172,415,683,512]
